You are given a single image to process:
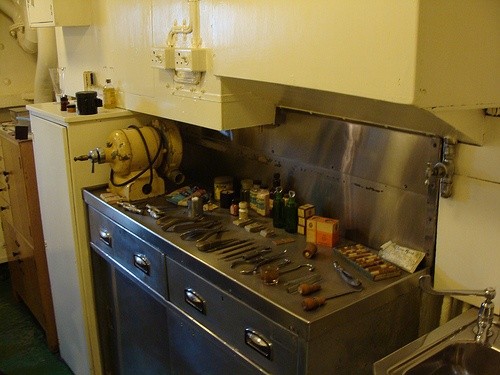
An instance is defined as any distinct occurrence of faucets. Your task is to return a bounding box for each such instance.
[418,274,496,346]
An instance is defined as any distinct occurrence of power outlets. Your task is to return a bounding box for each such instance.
[150,48,174,69]
[174,48,205,72]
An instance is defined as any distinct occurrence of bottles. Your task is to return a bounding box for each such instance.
[102,78,118,109]
[60,97,67,111]
[66,104,76,113]
[236,173,298,234]
[75,90,97,115]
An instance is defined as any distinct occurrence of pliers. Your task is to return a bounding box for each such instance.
[230,250,288,274]
[158,212,210,231]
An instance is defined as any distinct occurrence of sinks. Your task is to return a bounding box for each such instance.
[371,307,500,375]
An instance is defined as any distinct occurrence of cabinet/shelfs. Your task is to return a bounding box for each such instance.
[82,186,428,375]
[0,132,58,346]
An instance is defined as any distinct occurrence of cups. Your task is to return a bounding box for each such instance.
[48,67,66,104]
[187,196,202,218]
[220,189,235,208]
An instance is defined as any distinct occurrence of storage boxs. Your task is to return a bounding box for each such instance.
[298,203,339,247]
